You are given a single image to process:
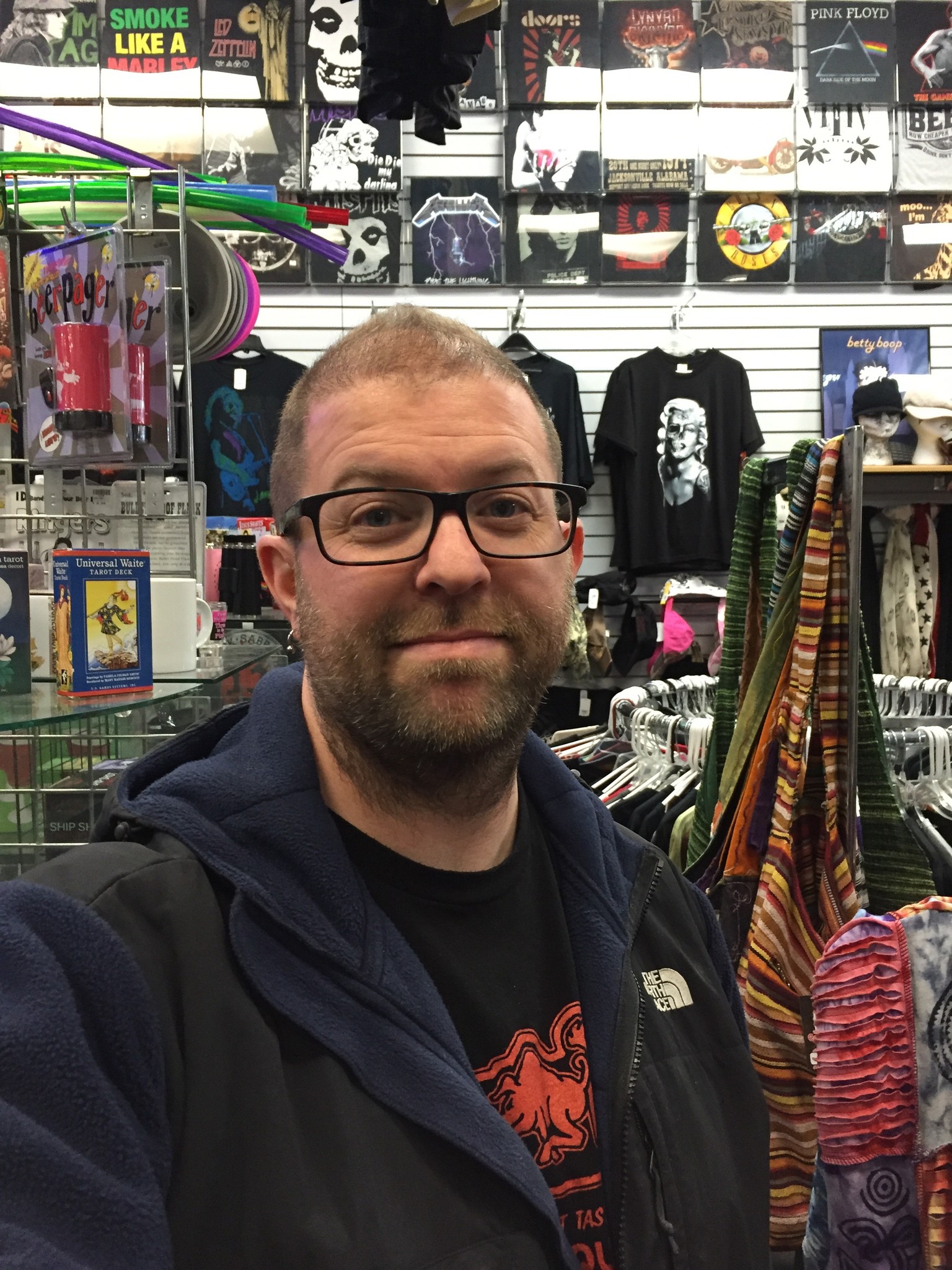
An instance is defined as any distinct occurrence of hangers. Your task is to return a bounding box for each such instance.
[554,674,952,867]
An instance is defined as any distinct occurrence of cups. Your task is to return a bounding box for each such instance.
[150,577,213,675]
[199,601,228,657]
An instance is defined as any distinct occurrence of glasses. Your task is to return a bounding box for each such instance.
[276,481,587,566]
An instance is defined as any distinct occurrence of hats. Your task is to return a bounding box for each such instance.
[901,381,952,419]
[851,380,907,426]
[564,570,727,681]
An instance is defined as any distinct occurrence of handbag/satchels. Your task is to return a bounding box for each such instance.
[681,825,937,1251]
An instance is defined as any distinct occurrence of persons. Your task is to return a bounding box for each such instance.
[0,302,772,1269]
